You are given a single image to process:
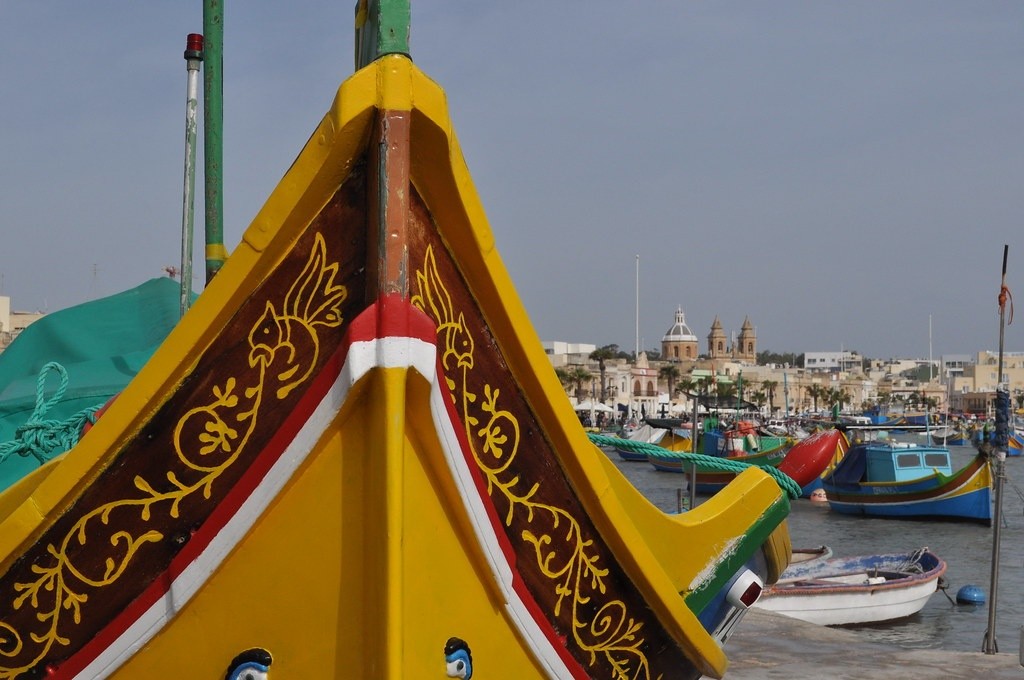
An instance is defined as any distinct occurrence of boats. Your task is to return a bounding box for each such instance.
[765,544,833,582]
[753,542,946,625]
[0,0,793,680]
[612,351,1023,522]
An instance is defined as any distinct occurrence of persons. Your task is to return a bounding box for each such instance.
[575,412,634,436]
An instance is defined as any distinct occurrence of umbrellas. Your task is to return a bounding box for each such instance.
[609,403,628,412]
[574,401,614,414]
